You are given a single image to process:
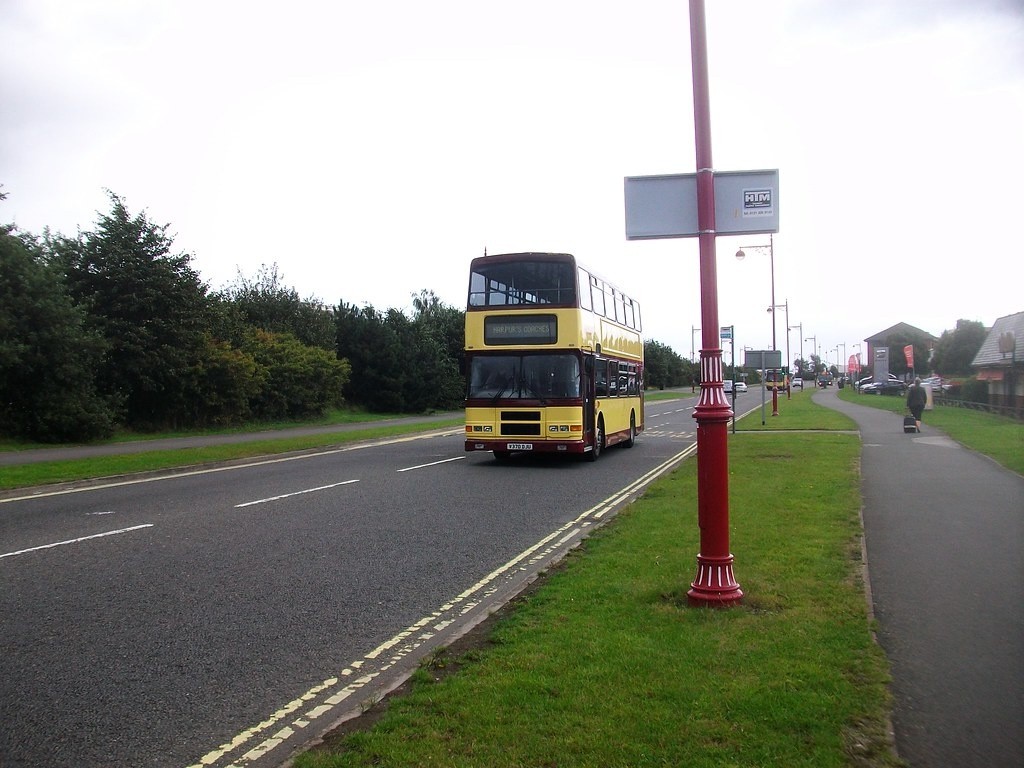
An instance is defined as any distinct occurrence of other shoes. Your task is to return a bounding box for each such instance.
[917,428,920,433]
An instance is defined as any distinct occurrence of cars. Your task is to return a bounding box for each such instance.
[734,382,748,392]
[855,374,909,396]
[819,374,833,385]
[919,376,951,393]
[792,376,804,388]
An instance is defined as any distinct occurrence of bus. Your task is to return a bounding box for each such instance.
[766,369,790,391]
[465,250,645,462]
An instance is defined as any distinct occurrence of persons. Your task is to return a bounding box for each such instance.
[906,378,927,433]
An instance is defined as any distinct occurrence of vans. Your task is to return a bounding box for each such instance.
[723,379,732,393]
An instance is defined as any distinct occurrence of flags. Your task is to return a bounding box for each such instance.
[855,353,861,373]
[848,355,855,373]
[904,345,913,368]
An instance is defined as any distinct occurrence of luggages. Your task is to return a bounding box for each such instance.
[904,408,916,433]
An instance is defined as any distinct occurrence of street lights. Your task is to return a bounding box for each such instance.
[830,349,839,373]
[819,346,821,362]
[805,332,818,387]
[837,341,846,376]
[788,323,804,394]
[767,298,791,400]
[735,234,779,416]
[721,338,733,361]
[853,344,862,376]
[744,346,753,352]
